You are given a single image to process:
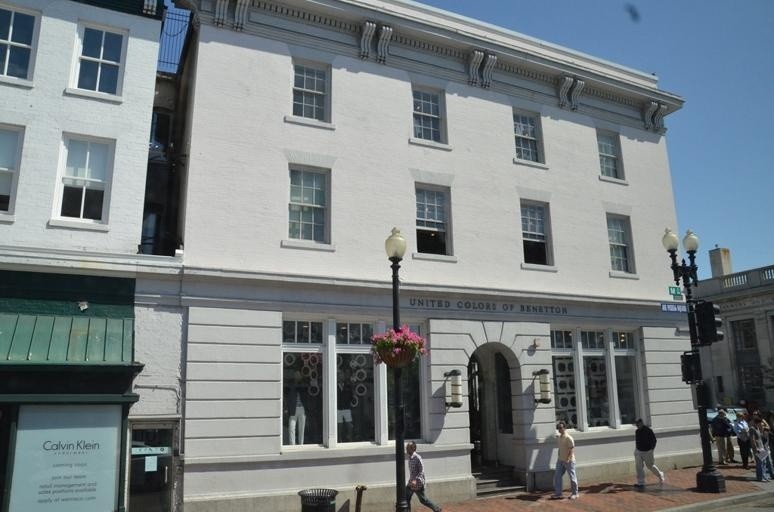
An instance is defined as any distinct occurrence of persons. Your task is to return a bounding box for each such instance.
[633,418,665,488]
[283,368,310,444]
[336,370,355,443]
[549,421,580,499]
[708,406,774,482]
[404,440,446,511]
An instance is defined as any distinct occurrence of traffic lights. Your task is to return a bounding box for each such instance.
[694,299,724,346]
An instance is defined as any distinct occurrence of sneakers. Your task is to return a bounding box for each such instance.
[719,459,738,465]
[659,472,664,482]
[636,482,644,486]
[552,493,578,499]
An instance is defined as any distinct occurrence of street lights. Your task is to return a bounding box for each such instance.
[385,227,410,512]
[660,227,727,493]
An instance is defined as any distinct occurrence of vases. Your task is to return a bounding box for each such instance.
[377,347,422,369]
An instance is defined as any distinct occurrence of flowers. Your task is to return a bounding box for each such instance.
[369,323,427,356]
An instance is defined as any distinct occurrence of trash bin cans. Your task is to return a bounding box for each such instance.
[298,489,338,512]
